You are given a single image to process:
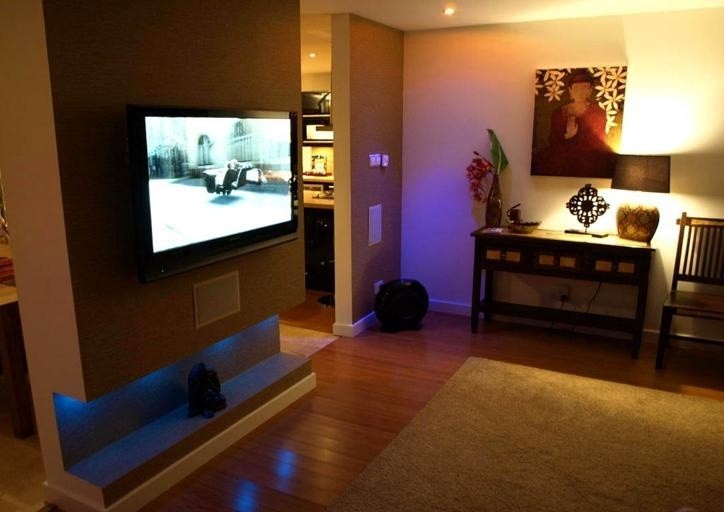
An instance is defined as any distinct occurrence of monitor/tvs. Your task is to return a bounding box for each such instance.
[127,105,300,281]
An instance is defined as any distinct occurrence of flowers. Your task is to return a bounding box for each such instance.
[465,129,509,203]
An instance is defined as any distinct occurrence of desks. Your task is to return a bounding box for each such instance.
[470,222,657,361]
[0,235,37,440]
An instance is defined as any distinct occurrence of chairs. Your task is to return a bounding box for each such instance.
[656,212,724,370]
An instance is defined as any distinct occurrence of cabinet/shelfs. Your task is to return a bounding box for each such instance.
[301,90,335,292]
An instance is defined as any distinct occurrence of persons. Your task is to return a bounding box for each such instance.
[544,71,606,176]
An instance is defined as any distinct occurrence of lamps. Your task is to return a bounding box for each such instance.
[610,155,670,242]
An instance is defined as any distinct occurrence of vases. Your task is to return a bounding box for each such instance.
[485,173,502,228]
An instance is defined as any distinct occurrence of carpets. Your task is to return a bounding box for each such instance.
[321,356,724,512]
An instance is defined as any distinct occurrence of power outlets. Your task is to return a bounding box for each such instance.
[557,286,569,302]
